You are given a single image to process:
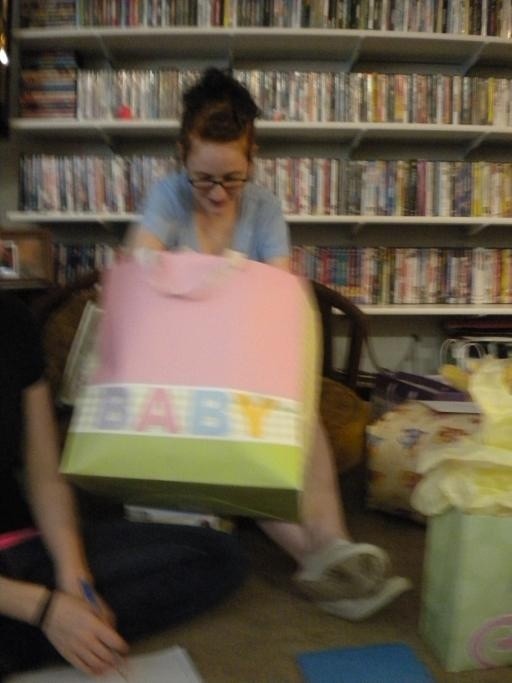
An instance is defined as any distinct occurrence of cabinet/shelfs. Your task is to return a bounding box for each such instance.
[7,0,511,315]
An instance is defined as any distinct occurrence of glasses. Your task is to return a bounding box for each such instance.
[181,151,255,191]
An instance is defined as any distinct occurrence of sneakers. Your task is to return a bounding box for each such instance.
[288,537,414,623]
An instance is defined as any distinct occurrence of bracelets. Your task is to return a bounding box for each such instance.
[35,587,56,629]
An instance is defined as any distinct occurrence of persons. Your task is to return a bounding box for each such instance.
[0,294,247,683]
[122,66,414,621]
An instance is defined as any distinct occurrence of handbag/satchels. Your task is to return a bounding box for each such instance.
[371,334,511,415]
[55,247,324,522]
[419,510,512,672]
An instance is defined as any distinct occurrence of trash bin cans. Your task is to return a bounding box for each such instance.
[416,468,512,671]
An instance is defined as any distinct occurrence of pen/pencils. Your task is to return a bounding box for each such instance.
[76,578,129,682]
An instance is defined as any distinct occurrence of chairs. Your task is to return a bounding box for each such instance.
[34,275,371,520]
